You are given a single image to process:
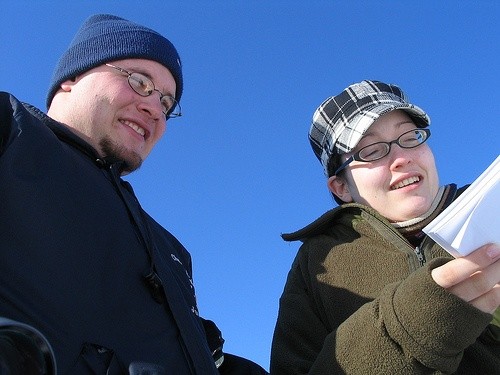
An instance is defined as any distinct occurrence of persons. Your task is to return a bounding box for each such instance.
[266,78,499,375]
[1,14,219,375]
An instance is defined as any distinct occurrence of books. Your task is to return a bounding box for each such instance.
[420,153,500,260]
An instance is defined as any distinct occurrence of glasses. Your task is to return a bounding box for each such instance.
[104,62,183,118]
[334,128,432,174]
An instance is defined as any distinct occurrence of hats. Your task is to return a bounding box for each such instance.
[45,12,183,121]
[309,78,431,179]
